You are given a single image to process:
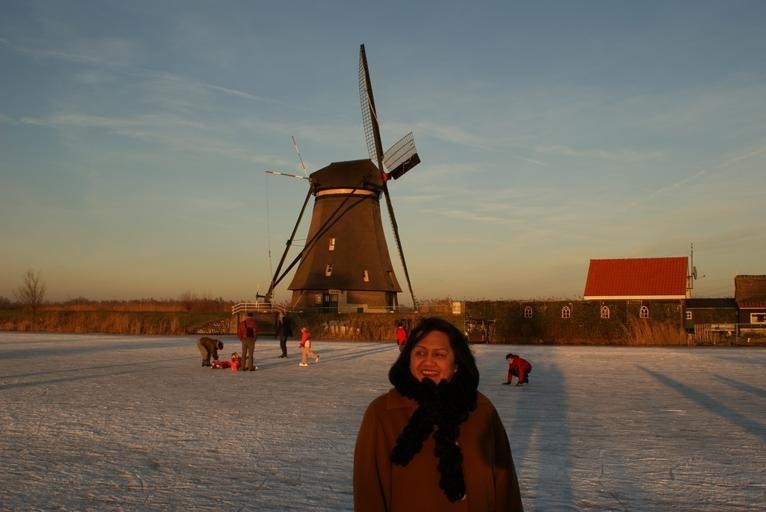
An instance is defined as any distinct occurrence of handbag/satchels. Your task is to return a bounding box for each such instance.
[246,327,253,337]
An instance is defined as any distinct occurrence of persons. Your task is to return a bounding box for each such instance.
[197,336,222,366]
[237,313,258,371]
[278,317,292,358]
[354,317,523,512]
[503,354,532,386]
[212,352,241,371]
[396,322,408,353]
[298,325,319,366]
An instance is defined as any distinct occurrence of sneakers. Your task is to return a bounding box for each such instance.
[299,362,308,367]
[280,355,287,358]
[201,363,211,367]
[316,355,320,363]
